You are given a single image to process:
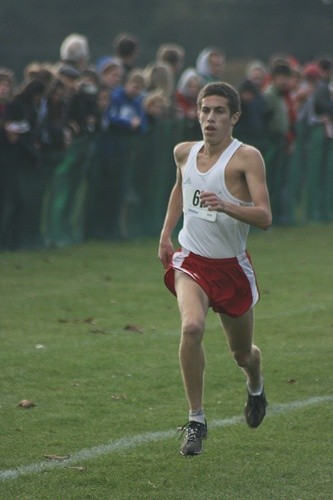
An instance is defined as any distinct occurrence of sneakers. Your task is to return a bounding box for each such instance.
[179,417,207,456]
[244,376,268,428]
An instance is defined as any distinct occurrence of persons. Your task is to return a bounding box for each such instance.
[0,34,333,252]
[157,81,272,456]
